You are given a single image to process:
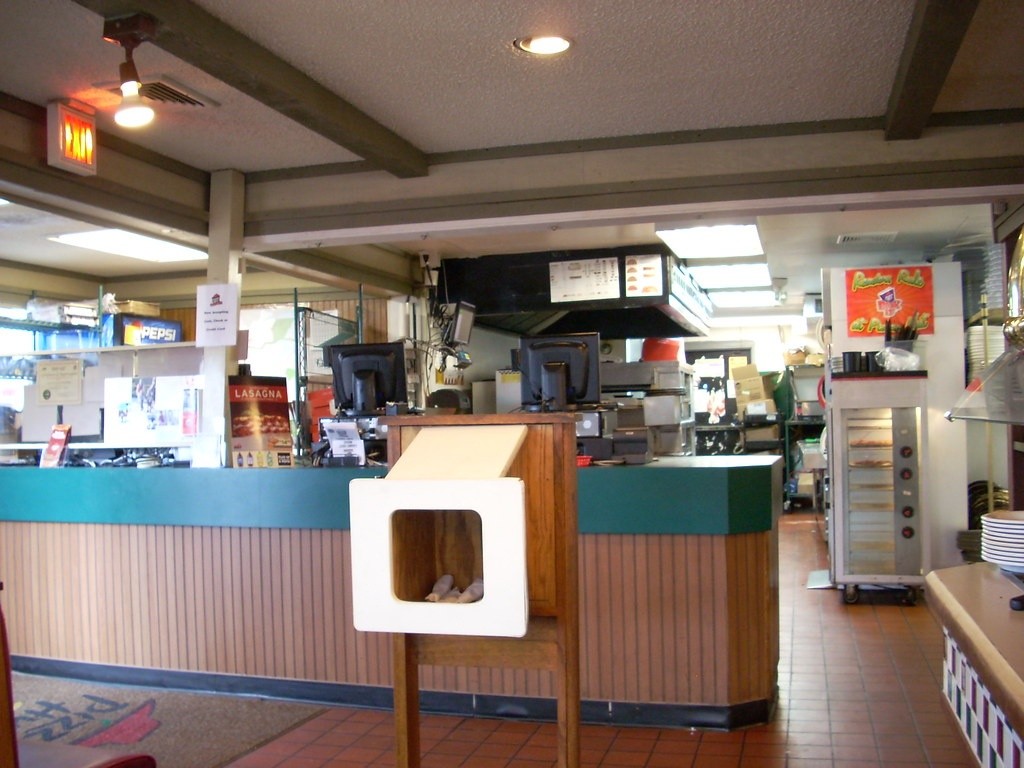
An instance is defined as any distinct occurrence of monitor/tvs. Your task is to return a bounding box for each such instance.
[450,298,477,345]
[521,332,602,412]
[330,342,408,417]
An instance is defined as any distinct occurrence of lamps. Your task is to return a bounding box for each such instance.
[114,40,155,129]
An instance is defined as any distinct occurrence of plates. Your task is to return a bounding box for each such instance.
[980,511,1024,574]
[968,326,1005,384]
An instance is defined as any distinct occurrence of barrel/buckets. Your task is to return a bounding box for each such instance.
[802,401,824,415]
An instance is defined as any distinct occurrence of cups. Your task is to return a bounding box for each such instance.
[866,351,882,372]
[842,351,862,373]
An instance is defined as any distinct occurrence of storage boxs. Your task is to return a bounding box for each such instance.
[745,427,827,492]
[117,299,162,317]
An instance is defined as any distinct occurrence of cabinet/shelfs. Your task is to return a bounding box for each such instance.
[742,407,824,499]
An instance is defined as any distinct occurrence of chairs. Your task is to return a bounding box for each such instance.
[0,580,156,768]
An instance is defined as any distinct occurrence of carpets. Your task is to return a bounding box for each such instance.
[13,674,332,768]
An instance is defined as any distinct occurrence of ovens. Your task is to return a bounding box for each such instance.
[831,370,931,603]
